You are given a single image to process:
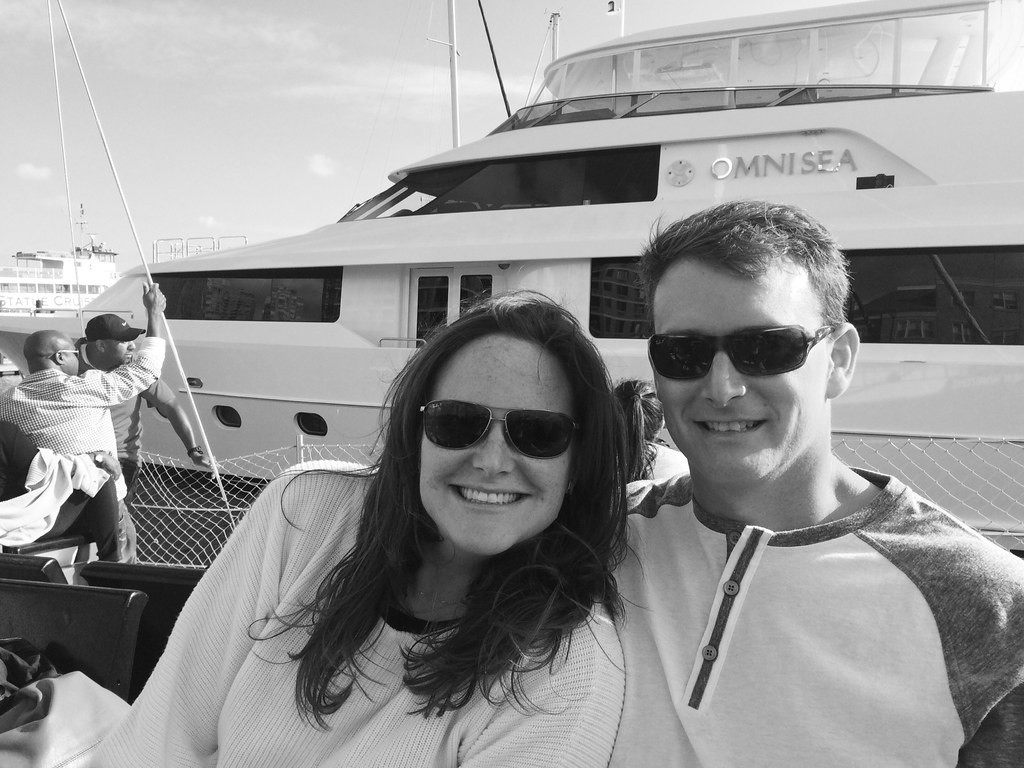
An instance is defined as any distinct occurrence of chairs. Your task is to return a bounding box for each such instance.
[0,577,149,704]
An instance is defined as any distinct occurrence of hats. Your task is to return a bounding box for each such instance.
[85,314,146,342]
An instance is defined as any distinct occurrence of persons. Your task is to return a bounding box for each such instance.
[613,379,666,482]
[611,200,1023,768]
[0,282,216,562]
[87,300,629,768]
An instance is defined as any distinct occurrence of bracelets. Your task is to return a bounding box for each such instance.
[188,446,203,457]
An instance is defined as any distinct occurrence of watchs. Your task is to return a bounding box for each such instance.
[92,451,104,467]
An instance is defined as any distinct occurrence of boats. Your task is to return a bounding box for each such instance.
[0,1,1024,565]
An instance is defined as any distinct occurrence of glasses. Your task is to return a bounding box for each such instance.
[48,348,80,359]
[420,400,579,459]
[647,323,835,381]
